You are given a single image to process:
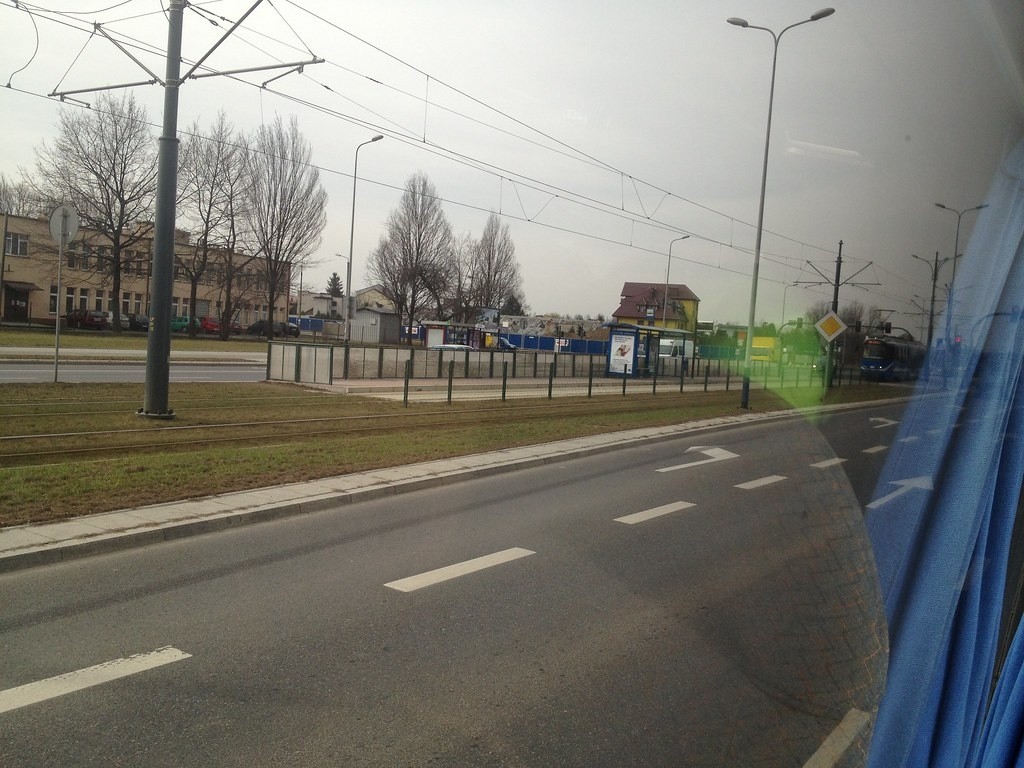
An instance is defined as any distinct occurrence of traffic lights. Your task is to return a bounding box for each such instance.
[955,335,961,348]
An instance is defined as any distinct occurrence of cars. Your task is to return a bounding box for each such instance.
[278,322,300,338]
[227,319,242,334]
[102,311,130,329]
[198,316,220,334]
[171,316,201,332]
[68,310,107,329]
[248,319,284,336]
[124,313,148,332]
[499,337,518,350]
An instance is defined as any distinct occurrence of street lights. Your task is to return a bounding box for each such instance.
[662,235,690,327]
[726,6,836,410]
[342,135,386,344]
[336,253,350,305]
[934,201,990,389]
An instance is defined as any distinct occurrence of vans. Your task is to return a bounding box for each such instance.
[658,338,686,358]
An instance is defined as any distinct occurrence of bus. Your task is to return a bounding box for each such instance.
[862,336,927,384]
